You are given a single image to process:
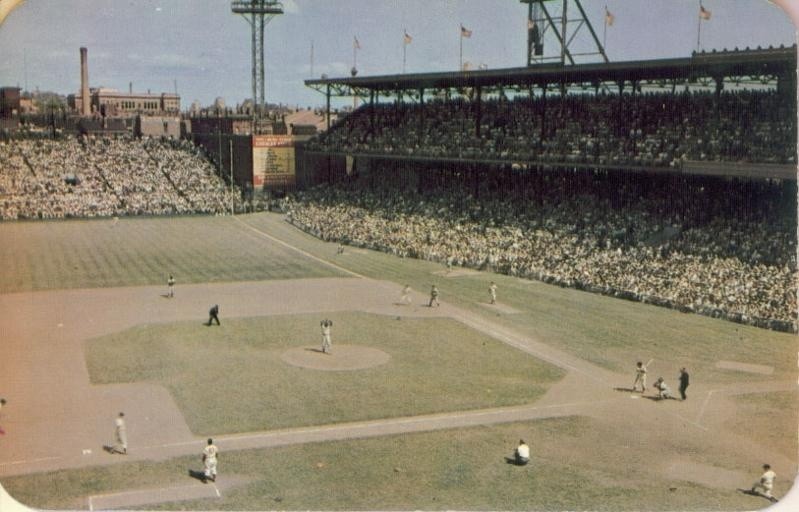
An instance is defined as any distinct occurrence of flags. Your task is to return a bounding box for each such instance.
[527,18,534,30]
[605,7,615,27]
[352,36,360,49]
[699,5,711,22]
[404,33,412,44]
[461,26,472,39]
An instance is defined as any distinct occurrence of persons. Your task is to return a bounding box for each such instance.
[166,273,176,300]
[320,319,335,353]
[748,463,779,503]
[109,412,129,455]
[272,86,799,333]
[0,120,272,222]
[207,301,222,328]
[632,361,691,401]
[200,438,220,484]
[514,438,530,464]
